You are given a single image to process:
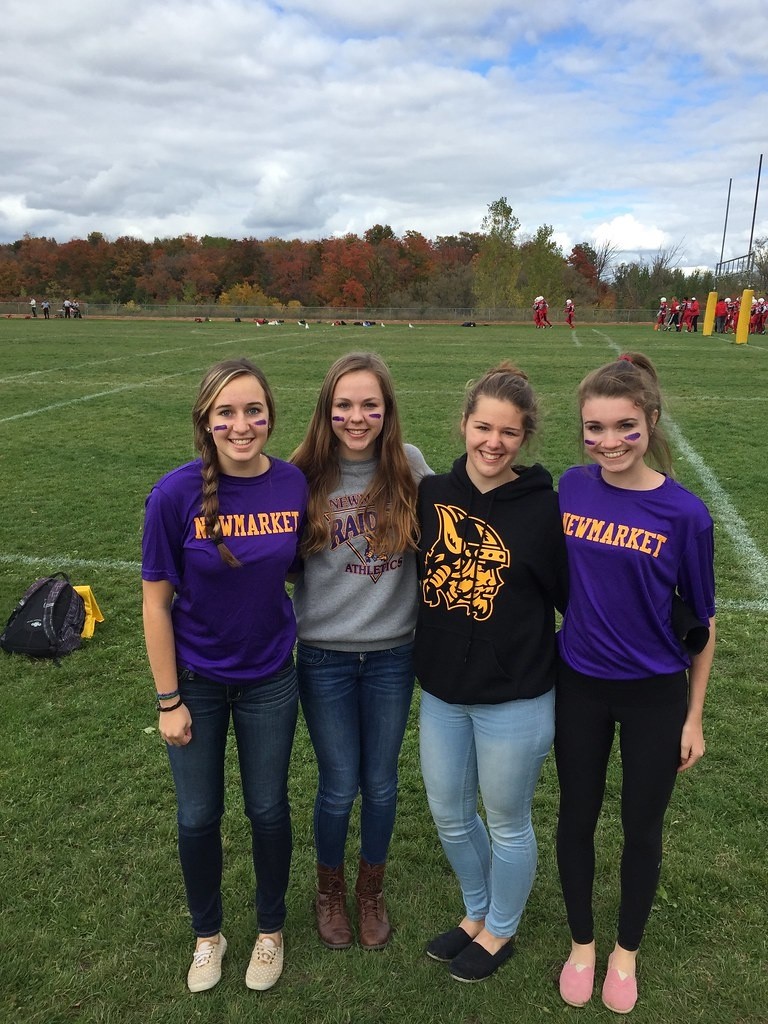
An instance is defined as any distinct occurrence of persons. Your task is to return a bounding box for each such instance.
[556,351,716,1012]
[686,297,700,333]
[41,299,50,319]
[29,297,36,317]
[73,300,82,318]
[63,298,73,318]
[142,357,308,992]
[716,296,768,335]
[564,300,575,328]
[668,296,681,332]
[657,297,673,331]
[416,367,710,984]
[533,296,553,329]
[679,297,692,332]
[288,352,435,950]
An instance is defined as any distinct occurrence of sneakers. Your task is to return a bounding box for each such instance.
[187,932,227,992]
[245,929,283,990]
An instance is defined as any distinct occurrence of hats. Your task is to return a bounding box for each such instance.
[690,298,696,300]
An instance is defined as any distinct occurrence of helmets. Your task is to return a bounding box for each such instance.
[566,299,571,303]
[752,299,757,305]
[758,298,765,304]
[761,297,766,302]
[725,298,731,304]
[534,296,544,302]
[660,297,666,302]
[735,297,739,302]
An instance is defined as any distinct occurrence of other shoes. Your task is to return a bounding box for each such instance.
[426,927,477,961]
[535,325,553,329]
[570,325,575,328]
[559,947,597,1007]
[657,327,766,335]
[448,932,514,983]
[602,953,637,1013]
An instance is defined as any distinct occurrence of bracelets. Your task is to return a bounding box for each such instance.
[157,700,182,712]
[158,689,179,700]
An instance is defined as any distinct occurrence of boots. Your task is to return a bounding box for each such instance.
[352,855,390,949]
[313,861,352,948]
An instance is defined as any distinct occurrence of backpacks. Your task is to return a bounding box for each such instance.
[0,572,86,659]
